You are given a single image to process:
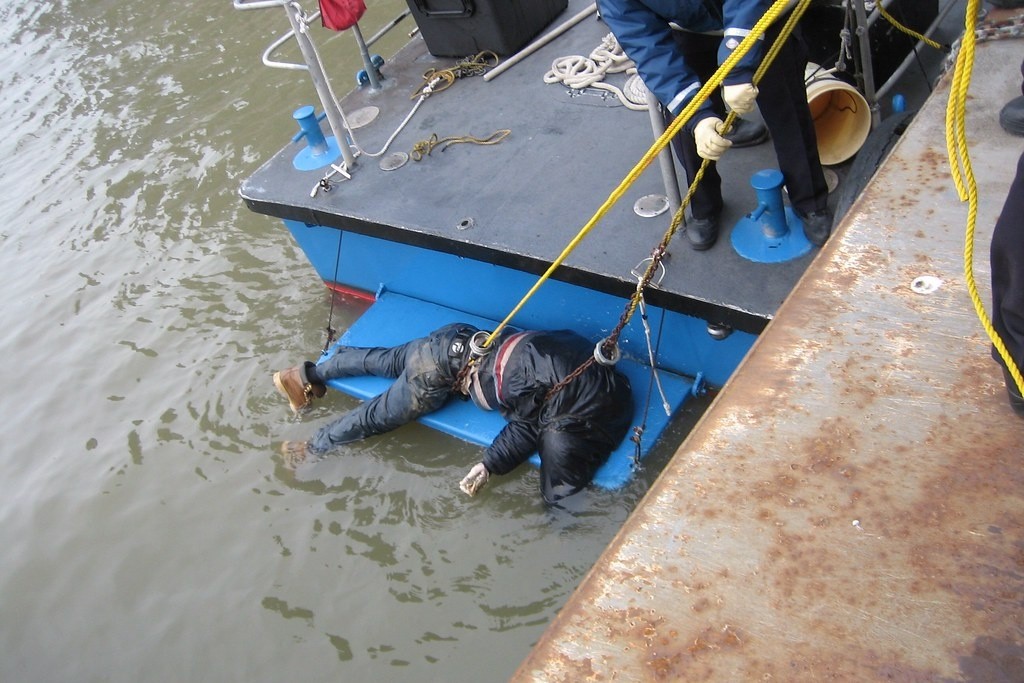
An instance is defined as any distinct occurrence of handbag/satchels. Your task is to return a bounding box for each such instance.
[318,0,367,31]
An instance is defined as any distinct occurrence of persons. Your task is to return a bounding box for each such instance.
[273,323,634,498]
[596,0,832,249]
[990,151,1024,409]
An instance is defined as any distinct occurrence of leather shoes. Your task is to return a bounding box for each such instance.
[684,209,718,251]
[721,112,769,148]
[791,204,834,247]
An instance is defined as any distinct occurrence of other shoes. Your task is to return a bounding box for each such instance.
[281,440,306,472]
[999,95,1024,136]
[272,361,327,416]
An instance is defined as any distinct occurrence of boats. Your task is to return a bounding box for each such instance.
[234,0,994,494]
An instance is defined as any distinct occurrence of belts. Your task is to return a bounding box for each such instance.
[448,328,474,378]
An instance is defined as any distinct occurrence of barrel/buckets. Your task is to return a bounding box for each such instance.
[803,62,872,164]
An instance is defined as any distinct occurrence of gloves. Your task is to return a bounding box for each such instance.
[723,82,759,116]
[694,116,733,161]
[459,462,490,498]
